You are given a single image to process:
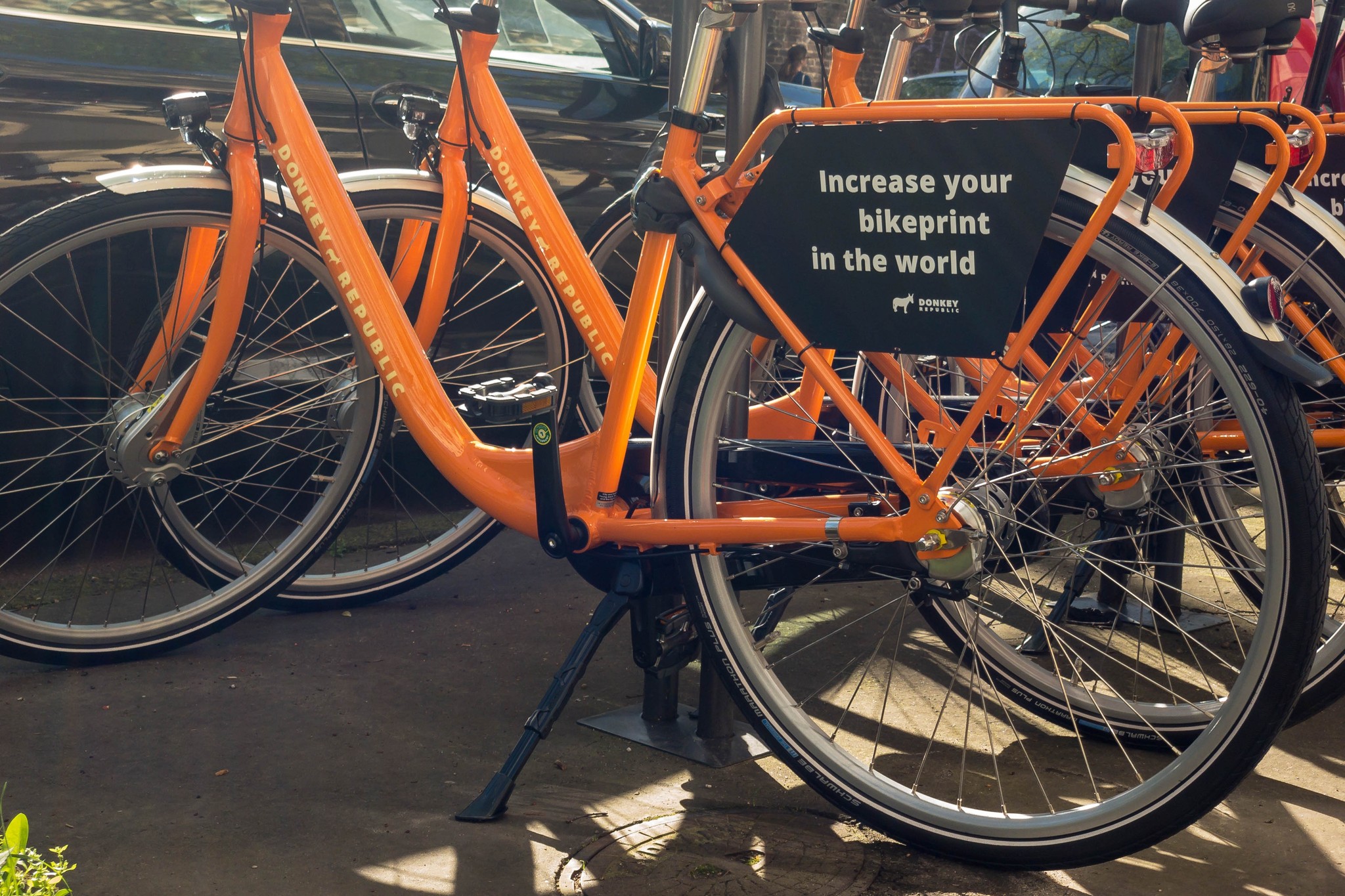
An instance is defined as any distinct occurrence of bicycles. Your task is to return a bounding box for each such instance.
[0,3,1344,877]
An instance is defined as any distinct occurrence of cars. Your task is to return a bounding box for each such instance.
[0,1,824,527]
